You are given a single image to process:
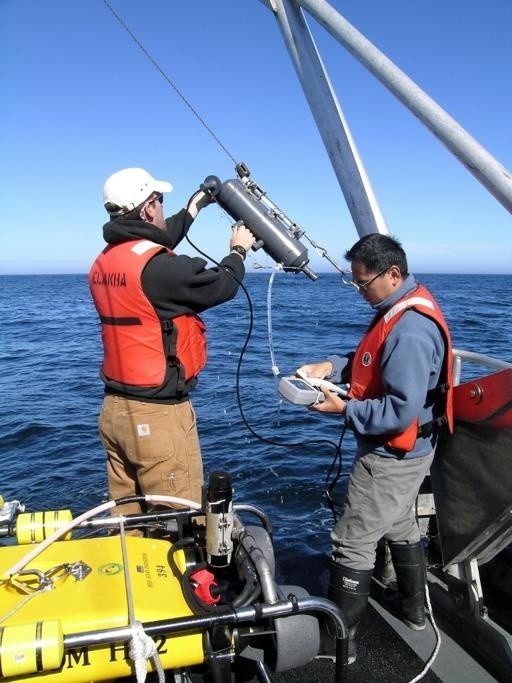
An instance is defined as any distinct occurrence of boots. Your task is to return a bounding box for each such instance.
[313,562,373,664]
[380,540,426,629]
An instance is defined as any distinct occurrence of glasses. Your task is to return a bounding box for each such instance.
[139,191,164,222]
[351,266,390,291]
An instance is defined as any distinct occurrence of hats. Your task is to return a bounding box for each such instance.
[103,166,173,211]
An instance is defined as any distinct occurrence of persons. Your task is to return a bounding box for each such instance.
[87,165,255,539]
[289,233,456,667]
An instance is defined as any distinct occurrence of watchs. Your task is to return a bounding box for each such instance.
[230,245,246,263]
